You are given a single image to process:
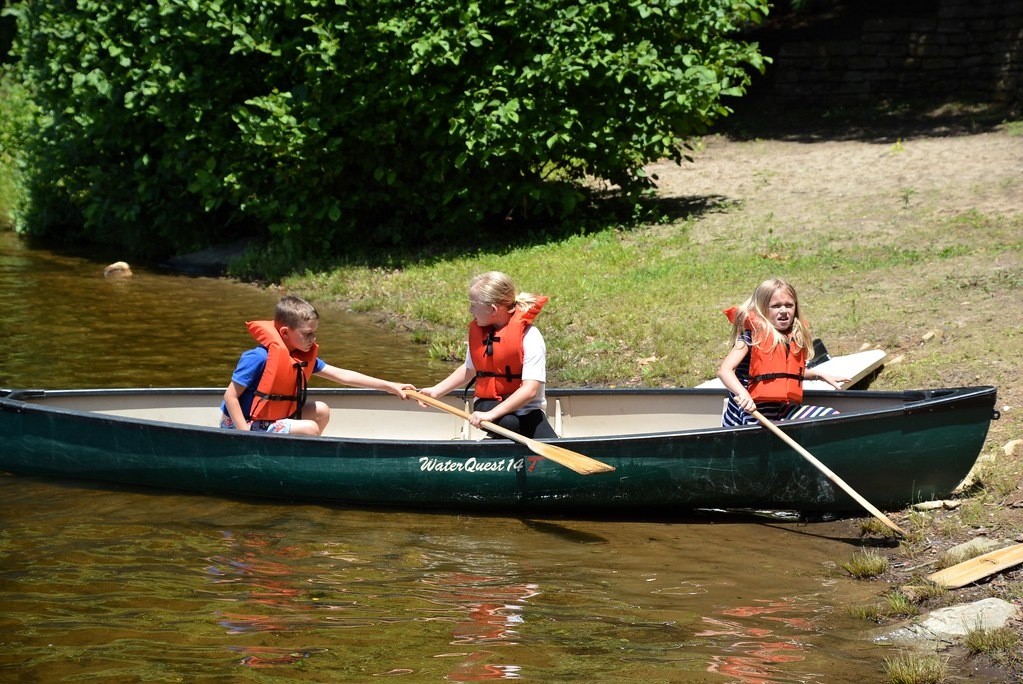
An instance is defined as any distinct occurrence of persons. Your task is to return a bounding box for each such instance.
[220,296,417,436]
[718,278,852,428]
[414,270,558,440]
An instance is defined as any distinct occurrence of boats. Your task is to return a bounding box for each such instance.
[0,384,1000,521]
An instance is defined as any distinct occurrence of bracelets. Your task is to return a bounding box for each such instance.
[816,369,820,380]
